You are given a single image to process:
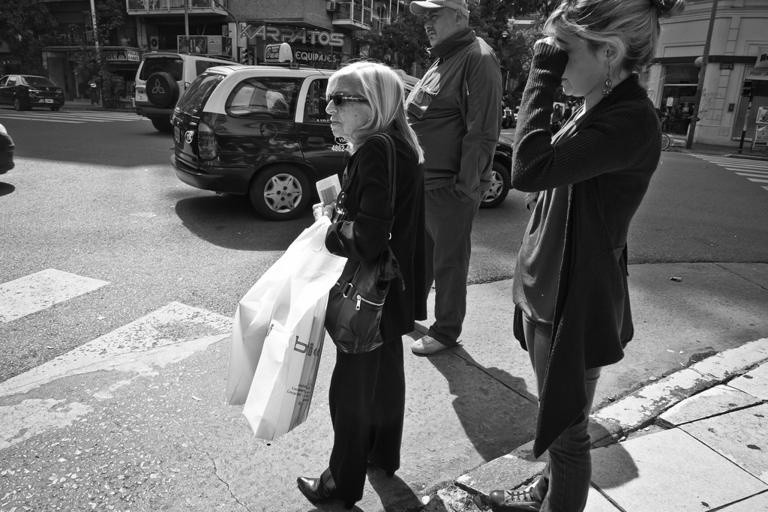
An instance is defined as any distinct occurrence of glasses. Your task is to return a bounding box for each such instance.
[324,94,367,105]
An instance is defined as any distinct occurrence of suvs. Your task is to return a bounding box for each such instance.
[129,50,244,134]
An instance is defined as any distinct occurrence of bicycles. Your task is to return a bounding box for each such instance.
[660,131,672,152]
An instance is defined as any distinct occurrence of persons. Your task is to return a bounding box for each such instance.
[297,62,428,509]
[404,0,504,357]
[492,0,688,512]
[552,100,581,126]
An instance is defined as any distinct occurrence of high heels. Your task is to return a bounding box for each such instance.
[297,475,357,511]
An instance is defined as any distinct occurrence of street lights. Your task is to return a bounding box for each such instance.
[741,80,752,97]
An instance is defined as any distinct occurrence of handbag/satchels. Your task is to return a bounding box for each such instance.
[325,244,405,354]
[223,216,347,441]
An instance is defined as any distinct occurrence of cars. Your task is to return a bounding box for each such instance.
[0,73,67,112]
[0,121,17,178]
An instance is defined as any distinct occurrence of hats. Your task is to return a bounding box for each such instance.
[408,0,470,18]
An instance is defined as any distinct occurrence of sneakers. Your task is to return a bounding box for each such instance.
[487,478,543,511]
[412,335,458,354]
